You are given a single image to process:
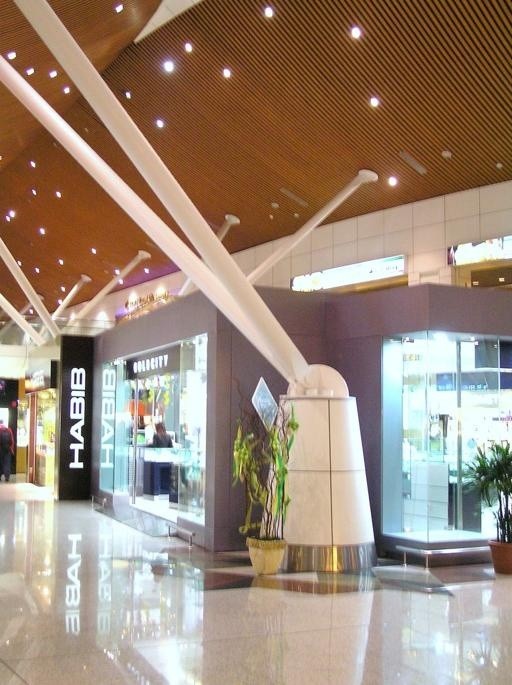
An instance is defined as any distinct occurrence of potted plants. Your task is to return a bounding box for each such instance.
[230,401,299,574]
[464,442,512,575]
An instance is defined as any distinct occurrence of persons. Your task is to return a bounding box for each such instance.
[0,420,15,481]
[148,422,173,448]
